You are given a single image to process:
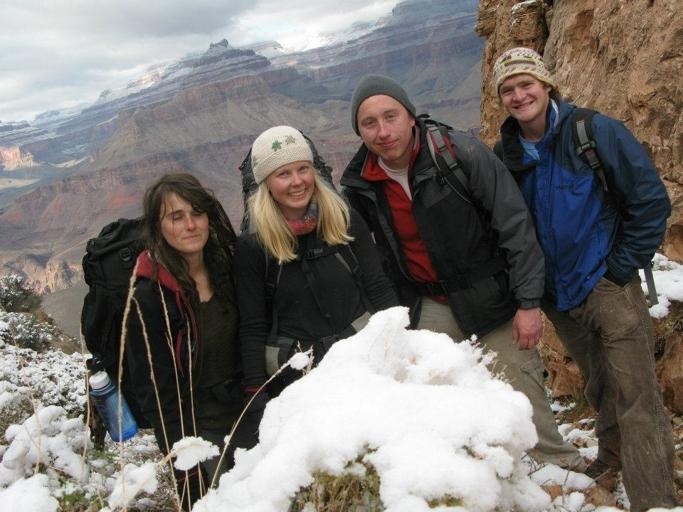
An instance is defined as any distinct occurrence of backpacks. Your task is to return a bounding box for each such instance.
[80,217,155,429]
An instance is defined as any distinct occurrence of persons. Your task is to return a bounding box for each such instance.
[122,172,285,512]
[327,70,589,480]
[483,44,683,510]
[228,120,405,452]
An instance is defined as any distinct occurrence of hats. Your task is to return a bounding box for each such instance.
[493,47,554,97]
[351,74,417,136]
[251,125,314,186]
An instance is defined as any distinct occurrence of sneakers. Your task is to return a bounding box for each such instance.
[583,458,622,481]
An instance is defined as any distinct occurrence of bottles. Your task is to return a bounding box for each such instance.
[87,370,139,443]
